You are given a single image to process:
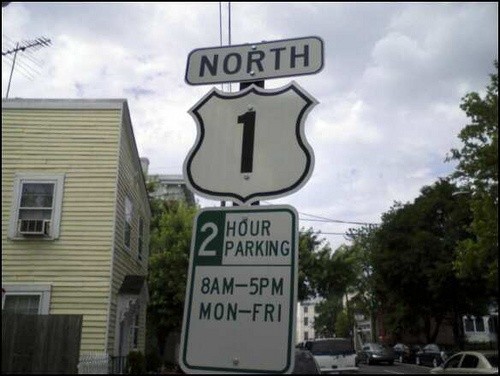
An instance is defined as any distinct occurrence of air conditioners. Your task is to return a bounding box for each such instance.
[17,220,48,238]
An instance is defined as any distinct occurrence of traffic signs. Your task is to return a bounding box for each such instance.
[178,204,299,375]
[184,34,325,86]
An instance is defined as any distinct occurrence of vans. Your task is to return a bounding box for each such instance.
[296,337,359,376]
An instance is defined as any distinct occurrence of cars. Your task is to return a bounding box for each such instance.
[429,349,500,375]
[414,344,446,367]
[294,348,319,375]
[392,341,420,364]
[356,343,392,367]
